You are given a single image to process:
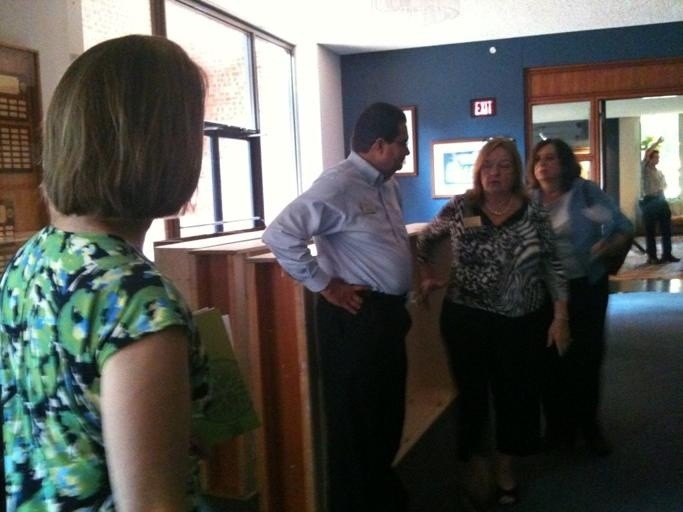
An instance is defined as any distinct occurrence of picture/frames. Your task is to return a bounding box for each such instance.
[430,137,486,198]
[389,104,418,176]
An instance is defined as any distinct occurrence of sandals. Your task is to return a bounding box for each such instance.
[495,482,520,508]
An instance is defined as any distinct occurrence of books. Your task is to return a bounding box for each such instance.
[192,304,265,448]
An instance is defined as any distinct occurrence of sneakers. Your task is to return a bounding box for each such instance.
[592,434,614,457]
[548,430,576,461]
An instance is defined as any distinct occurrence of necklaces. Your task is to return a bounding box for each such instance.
[480,197,515,215]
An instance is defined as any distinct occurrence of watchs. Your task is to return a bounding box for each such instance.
[554,313,571,323]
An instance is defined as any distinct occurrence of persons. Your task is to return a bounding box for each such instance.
[640,137,681,265]
[2,34,215,510]
[418,136,568,508]
[525,140,633,456]
[263,100,414,510]
